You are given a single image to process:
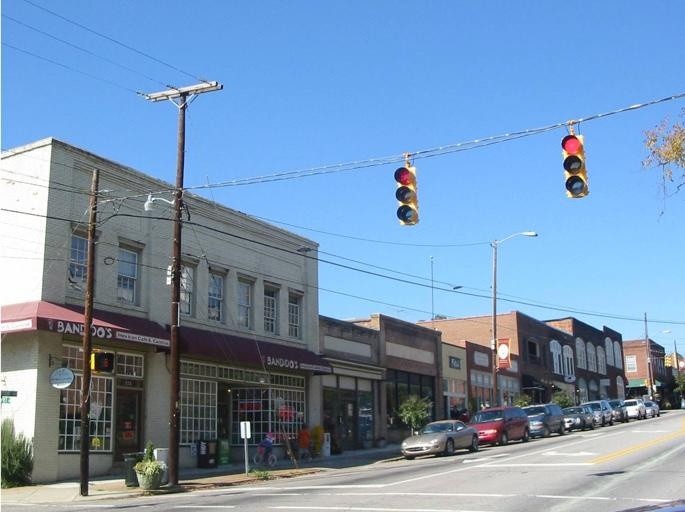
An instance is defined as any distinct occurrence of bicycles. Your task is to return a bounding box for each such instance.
[254,445,276,467]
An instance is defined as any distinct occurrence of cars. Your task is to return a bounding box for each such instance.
[400,399,659,459]
[359,409,372,421]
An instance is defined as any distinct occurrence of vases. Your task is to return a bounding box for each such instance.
[364,439,374,449]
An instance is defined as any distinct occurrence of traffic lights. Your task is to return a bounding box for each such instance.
[394,167,418,225]
[90,352,114,375]
[560,135,587,198]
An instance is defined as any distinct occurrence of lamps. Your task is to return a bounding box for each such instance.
[152,346,169,354]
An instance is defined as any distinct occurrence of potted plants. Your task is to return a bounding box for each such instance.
[375,436,386,448]
[133,440,168,492]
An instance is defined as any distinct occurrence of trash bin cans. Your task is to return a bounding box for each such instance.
[681,399,685,408]
[320,433,331,457]
[123,452,146,487]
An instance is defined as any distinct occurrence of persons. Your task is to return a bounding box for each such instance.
[460,407,470,423]
[504,395,508,405]
[258,431,274,461]
[450,405,460,419]
[296,423,313,462]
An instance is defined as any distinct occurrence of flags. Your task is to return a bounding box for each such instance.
[496,338,511,370]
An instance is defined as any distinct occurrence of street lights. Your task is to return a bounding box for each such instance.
[647,331,671,400]
[492,231,537,407]
[145,192,184,485]
